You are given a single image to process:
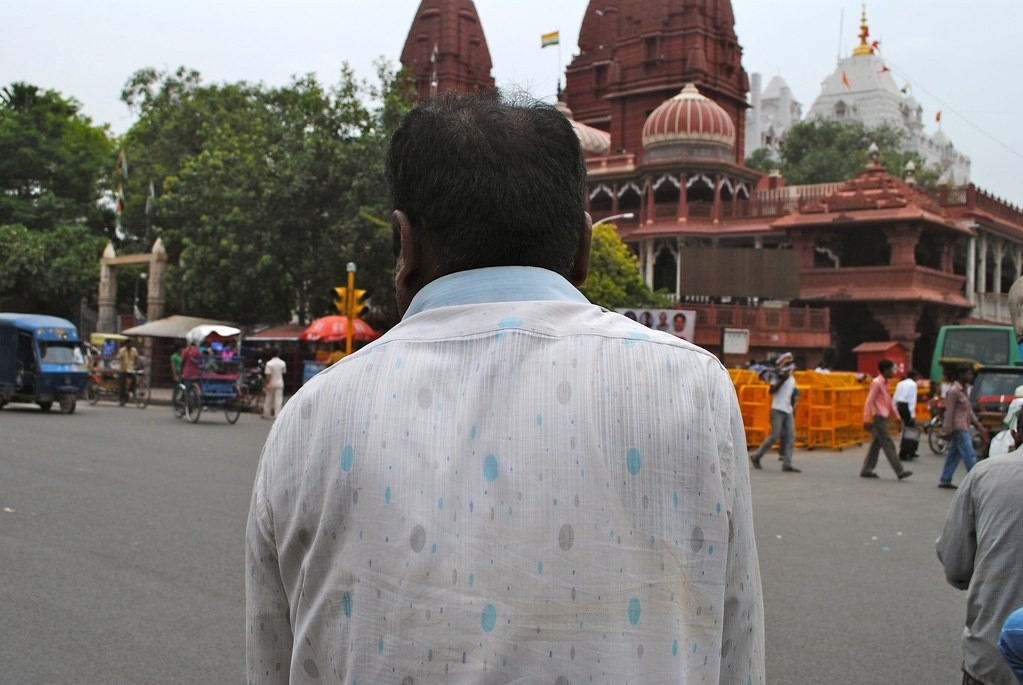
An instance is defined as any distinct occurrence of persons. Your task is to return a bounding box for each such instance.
[938,366,988,489]
[988,384,1023,459]
[625,312,685,331]
[815,363,832,372]
[323,342,345,365]
[859,358,913,480]
[893,369,921,460]
[102,339,116,368]
[262,348,288,419]
[171,343,202,418]
[935,443,1023,685]
[750,351,802,472]
[245,85,765,685]
[116,339,140,407]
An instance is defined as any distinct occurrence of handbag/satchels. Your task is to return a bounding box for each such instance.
[903,427,919,441]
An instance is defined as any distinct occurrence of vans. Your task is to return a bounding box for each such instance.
[924,323,1023,434]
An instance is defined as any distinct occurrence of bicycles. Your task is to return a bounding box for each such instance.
[926,394,952,455]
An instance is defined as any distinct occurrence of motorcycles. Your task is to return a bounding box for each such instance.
[0,312,92,415]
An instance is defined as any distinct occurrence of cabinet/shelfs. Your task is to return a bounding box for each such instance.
[728,368,931,452]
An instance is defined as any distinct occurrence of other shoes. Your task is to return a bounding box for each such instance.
[938,483,957,489]
[860,471,876,478]
[749,454,760,468]
[782,466,800,473]
[898,471,912,479]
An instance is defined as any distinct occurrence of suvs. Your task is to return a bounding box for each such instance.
[967,363,1023,451]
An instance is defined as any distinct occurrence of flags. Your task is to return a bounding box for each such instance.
[541,31,559,48]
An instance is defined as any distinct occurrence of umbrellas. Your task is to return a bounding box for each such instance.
[299,315,382,342]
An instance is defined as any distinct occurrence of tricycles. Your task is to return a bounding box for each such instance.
[82,332,152,409]
[172,354,247,424]
